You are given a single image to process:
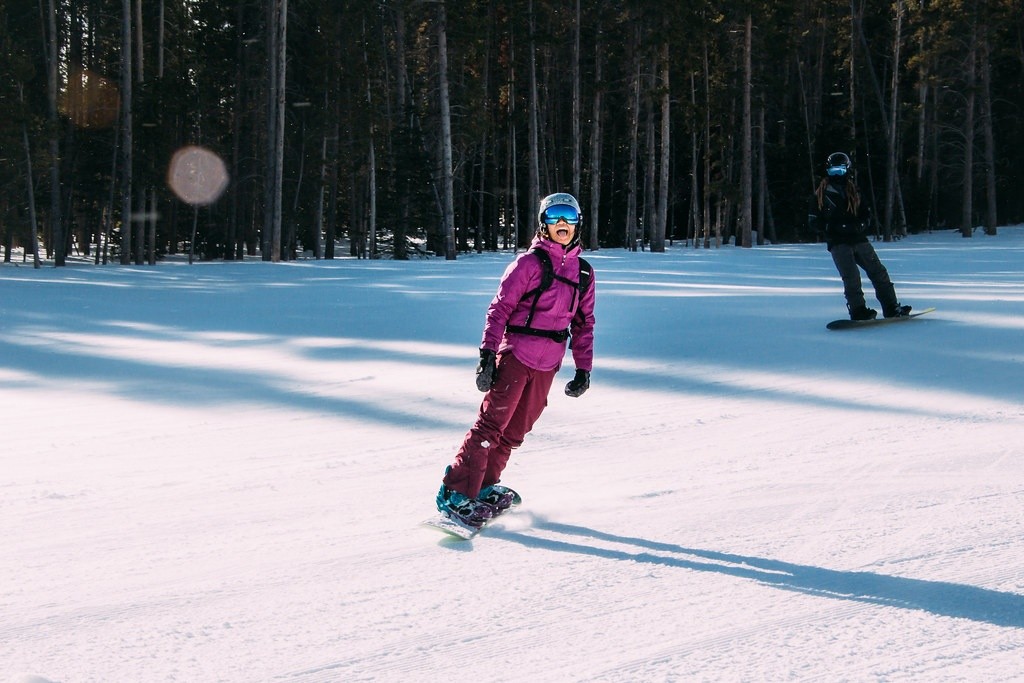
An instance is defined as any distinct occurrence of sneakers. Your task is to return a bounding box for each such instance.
[437,489,492,528]
[477,490,513,508]
[850,306,877,320]
[883,302,912,317]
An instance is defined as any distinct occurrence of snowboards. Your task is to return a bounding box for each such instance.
[826,307,936,330]
[421,483,522,542]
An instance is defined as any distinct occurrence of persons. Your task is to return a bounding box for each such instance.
[436,193,595,528]
[807,152,912,321]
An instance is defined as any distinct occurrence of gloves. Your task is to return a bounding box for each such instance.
[564,369,590,397]
[475,349,497,392]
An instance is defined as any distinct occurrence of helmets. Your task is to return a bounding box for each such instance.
[827,152,850,169]
[538,192,584,227]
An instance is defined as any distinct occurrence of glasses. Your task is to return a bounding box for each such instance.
[826,165,847,176]
[542,204,580,225]
